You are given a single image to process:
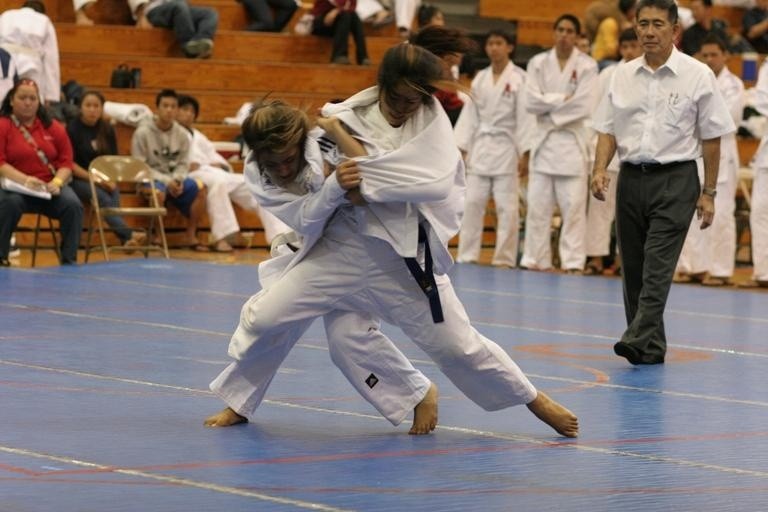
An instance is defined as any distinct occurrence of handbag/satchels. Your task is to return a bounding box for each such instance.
[111,63,142,88]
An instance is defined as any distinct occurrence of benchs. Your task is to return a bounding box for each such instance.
[0,0,768,250]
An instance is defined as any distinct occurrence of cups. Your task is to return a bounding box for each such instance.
[132,67,141,88]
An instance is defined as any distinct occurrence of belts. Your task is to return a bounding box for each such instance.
[624,163,675,176]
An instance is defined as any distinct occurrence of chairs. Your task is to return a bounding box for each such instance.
[7,213,67,265]
[85,155,167,263]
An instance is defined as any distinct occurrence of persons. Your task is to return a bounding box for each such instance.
[66,89,148,254]
[590,0,767,288]
[71,0,462,83]
[590,0,738,365]
[239,88,438,436]
[525,13,598,276]
[177,94,258,251]
[451,28,529,269]
[0,77,84,267]
[204,24,578,440]
[129,89,209,250]
[0,0,61,110]
